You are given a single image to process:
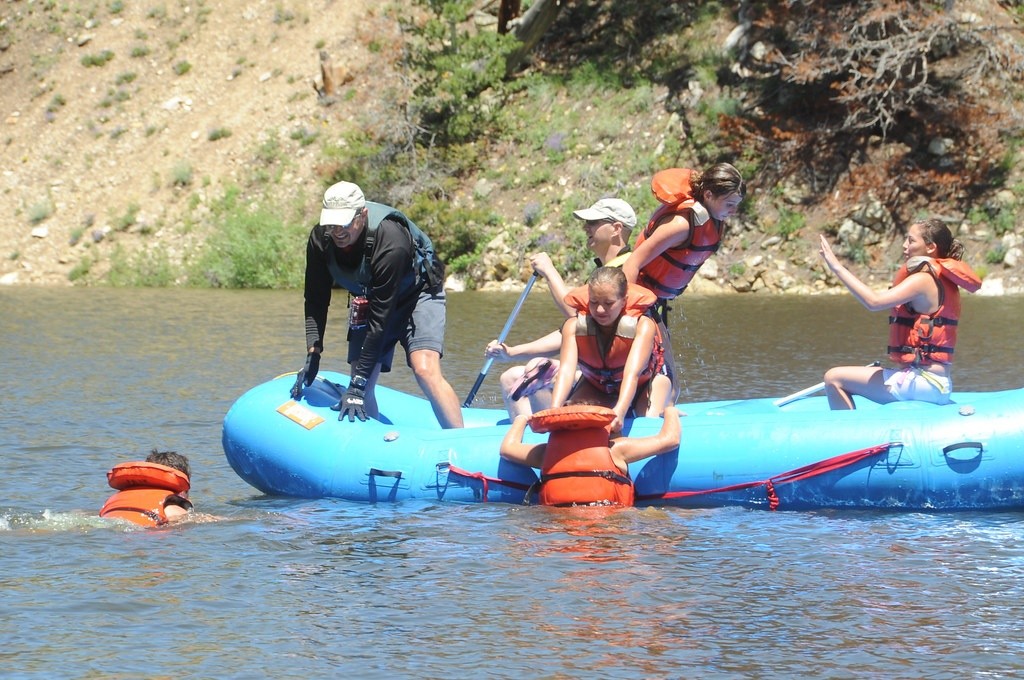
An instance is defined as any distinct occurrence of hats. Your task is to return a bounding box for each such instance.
[319,179,367,227]
[572,198,637,233]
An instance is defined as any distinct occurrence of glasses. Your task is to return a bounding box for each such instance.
[585,219,626,230]
[326,211,362,231]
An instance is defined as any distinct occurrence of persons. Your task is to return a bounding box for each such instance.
[621,164,747,304]
[498,398,687,504]
[550,265,672,430]
[290,180,465,430]
[484,197,678,423]
[819,218,982,412]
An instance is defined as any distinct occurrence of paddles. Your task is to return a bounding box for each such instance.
[464,267,542,409]
[771,359,882,409]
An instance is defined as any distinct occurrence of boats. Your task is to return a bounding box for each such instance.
[221,371,1024,515]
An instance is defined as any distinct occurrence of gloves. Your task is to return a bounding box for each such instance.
[291,352,321,400]
[330,383,371,422]
